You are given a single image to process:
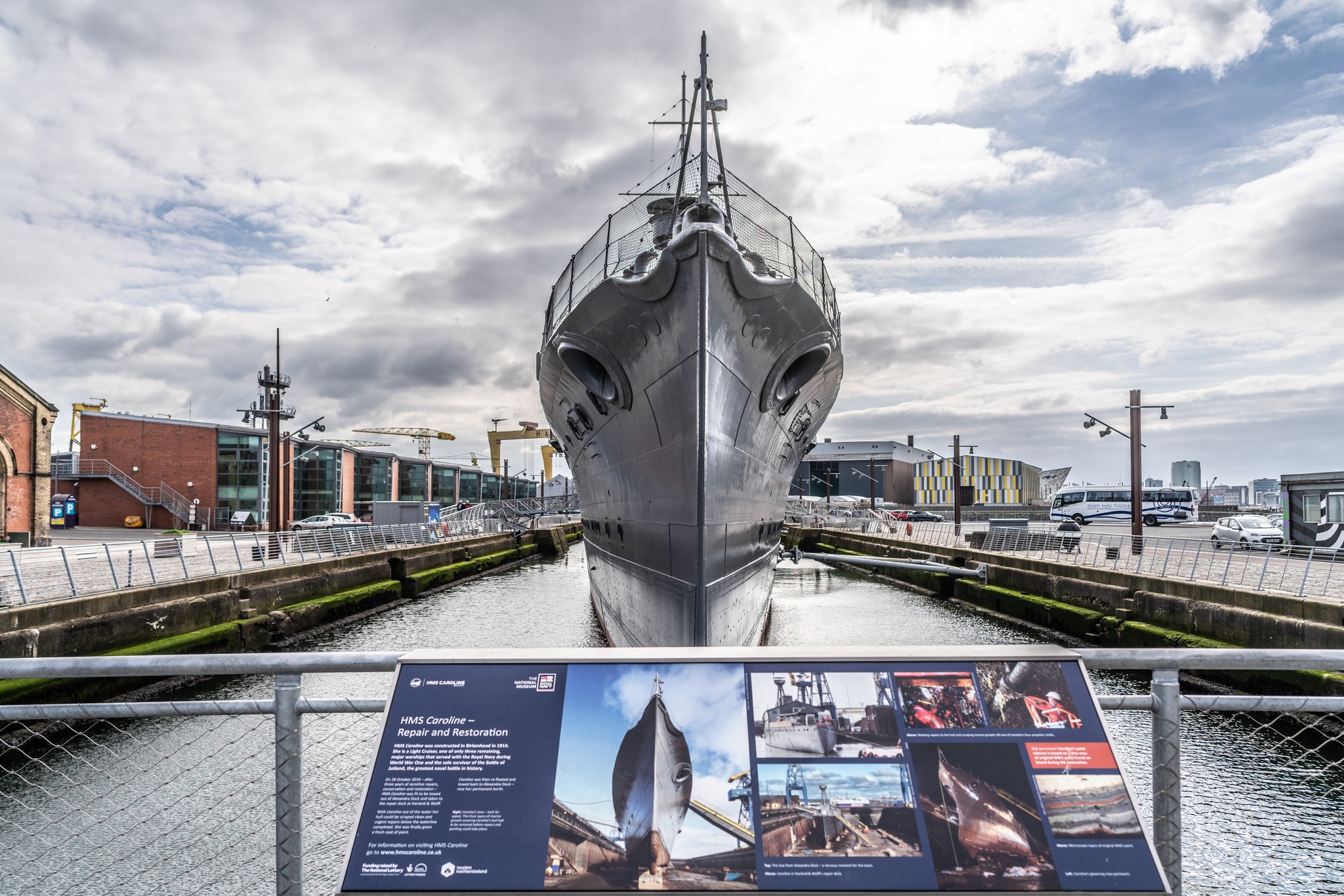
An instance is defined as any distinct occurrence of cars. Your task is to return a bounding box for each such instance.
[906,511,944,523]
[832,510,853,517]
[897,510,915,521]
[1211,513,1283,552]
[866,510,903,520]
[289,513,361,533]
[819,510,825,514]
[357,514,373,525]
[532,509,581,515]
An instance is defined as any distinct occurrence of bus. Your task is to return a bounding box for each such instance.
[1047,485,1198,527]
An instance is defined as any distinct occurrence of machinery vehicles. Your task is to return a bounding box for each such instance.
[1199,477,1217,506]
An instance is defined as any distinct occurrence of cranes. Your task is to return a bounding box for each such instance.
[318,439,391,448]
[352,428,456,460]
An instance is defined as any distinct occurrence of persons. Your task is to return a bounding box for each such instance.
[822,716,826,725]
[831,509,834,514]
[464,500,472,520]
[825,508,827,512]
[1039,691,1070,722]
[826,715,831,725]
[908,686,945,728]
[1270,506,1271,511]
[456,500,466,521]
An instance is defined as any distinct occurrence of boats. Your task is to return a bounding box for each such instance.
[760,666,842,756]
[611,679,693,875]
[918,745,1054,882]
[535,29,845,586]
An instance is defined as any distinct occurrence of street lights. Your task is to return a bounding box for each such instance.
[812,467,840,515]
[928,435,978,535]
[791,475,810,508]
[1084,390,1174,555]
[851,458,888,519]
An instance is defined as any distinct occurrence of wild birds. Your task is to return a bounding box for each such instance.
[324,297,330,301]
